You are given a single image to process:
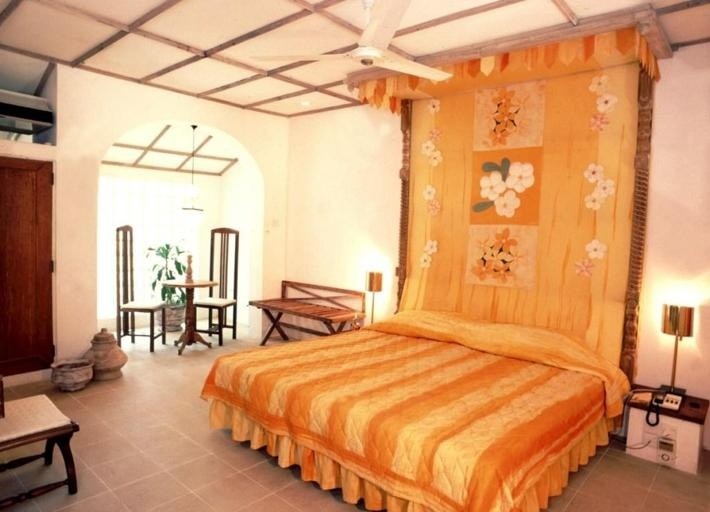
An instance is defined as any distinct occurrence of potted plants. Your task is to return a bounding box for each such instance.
[146,243,188,333]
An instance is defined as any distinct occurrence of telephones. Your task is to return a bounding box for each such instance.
[645,385,687,427]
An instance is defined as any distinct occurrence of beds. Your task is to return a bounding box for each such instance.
[202,281,643,511]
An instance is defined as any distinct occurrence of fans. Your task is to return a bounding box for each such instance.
[249,0,453,82]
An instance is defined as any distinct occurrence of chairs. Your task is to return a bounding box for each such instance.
[193,227,240,346]
[116,225,166,352]
[248,280,365,347]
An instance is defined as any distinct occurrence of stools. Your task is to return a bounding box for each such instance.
[0,393,80,511]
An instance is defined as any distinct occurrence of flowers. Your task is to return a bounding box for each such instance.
[420,254,432,267]
[575,238,607,278]
[473,158,510,212]
[428,99,441,113]
[422,130,443,168]
[587,75,618,134]
[494,190,521,218]
[425,240,439,255]
[505,162,536,193]
[471,226,534,283]
[583,163,618,211]
[422,184,441,216]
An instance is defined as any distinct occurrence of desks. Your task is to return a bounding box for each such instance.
[158,280,219,356]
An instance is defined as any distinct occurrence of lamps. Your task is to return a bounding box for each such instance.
[659,303,695,397]
[368,271,382,324]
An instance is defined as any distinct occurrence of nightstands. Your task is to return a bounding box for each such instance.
[625,385,710,475]
